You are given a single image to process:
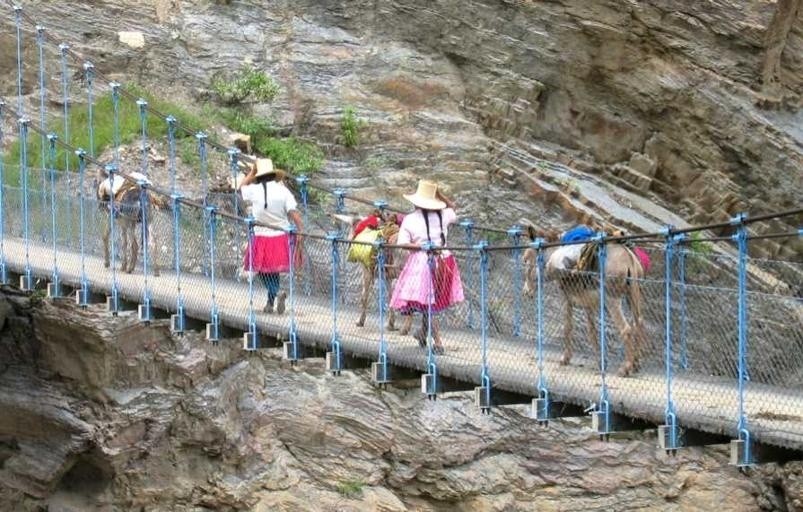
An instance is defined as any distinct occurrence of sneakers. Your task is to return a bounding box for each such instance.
[275,290,287,314]
[413,329,445,355]
[262,301,274,314]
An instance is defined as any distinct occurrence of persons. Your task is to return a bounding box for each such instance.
[388,180,467,354]
[238,157,305,315]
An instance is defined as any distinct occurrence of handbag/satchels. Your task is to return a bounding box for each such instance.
[541,243,587,284]
[630,248,652,278]
[344,225,386,271]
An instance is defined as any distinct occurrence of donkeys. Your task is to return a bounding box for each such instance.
[83,168,161,277]
[330,211,416,336]
[521,226,648,378]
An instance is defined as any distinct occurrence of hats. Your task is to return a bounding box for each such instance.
[247,157,285,184]
[402,177,447,211]
